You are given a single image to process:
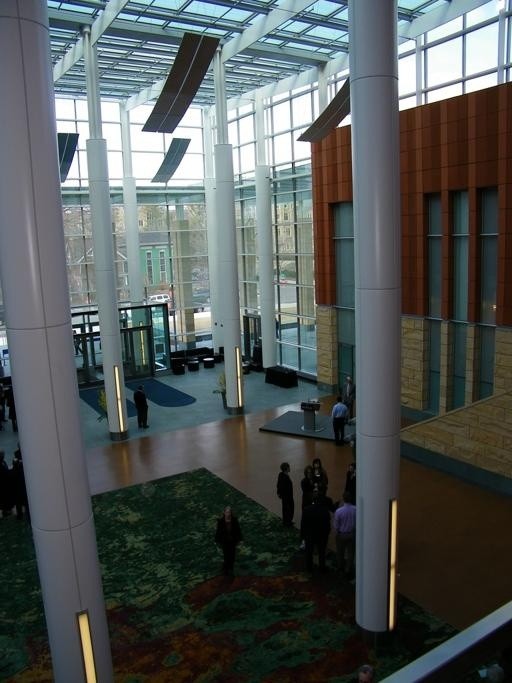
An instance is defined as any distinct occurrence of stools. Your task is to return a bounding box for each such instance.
[213,354,221,363]
[203,358,215,368]
[174,363,185,374]
[188,360,199,371]
[242,360,251,374]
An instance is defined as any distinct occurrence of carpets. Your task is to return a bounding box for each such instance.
[126,378,196,407]
[79,387,138,418]
[0,468,512,683]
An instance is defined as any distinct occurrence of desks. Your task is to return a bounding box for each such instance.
[265,366,298,388]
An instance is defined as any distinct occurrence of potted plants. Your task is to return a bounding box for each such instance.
[212,369,227,409]
[95,389,108,422]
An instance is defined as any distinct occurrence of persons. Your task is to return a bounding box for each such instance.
[341,375,355,426]
[276,457,359,569]
[214,505,244,585]
[348,664,377,683]
[329,395,348,446]
[133,385,150,428]
[0,380,30,523]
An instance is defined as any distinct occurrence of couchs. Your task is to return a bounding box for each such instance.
[171,347,214,368]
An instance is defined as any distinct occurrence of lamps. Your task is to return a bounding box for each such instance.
[75,608,98,683]
[387,496,399,632]
